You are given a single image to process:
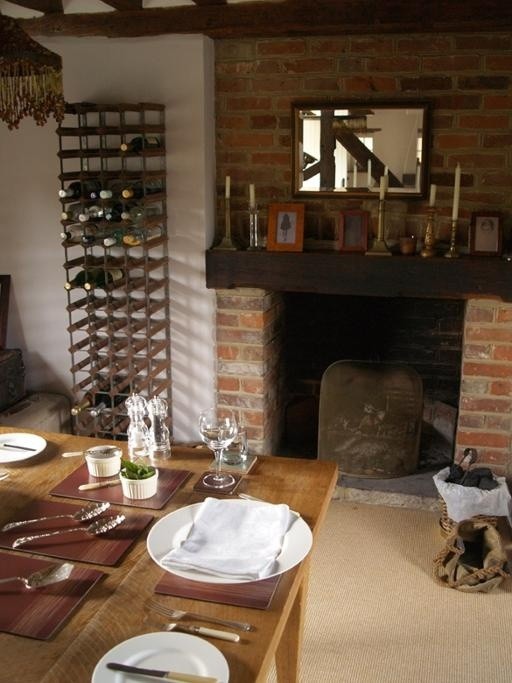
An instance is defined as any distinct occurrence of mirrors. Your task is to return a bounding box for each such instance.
[288,98,432,200]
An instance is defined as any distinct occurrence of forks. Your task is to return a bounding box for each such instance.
[143,598,251,631]
[144,615,239,643]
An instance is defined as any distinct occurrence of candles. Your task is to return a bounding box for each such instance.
[429,184,437,209]
[367,159,372,186]
[352,159,358,187]
[379,175,387,202]
[225,175,231,199]
[249,184,256,210]
[415,165,421,192]
[452,161,461,221]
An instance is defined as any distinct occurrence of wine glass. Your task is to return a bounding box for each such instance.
[198,407,238,486]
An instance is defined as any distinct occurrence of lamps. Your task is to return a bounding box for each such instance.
[0,15,66,131]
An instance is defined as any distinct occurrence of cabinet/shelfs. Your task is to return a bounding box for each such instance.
[55,101,174,445]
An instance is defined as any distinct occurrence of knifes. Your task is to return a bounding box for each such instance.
[106,662,217,683]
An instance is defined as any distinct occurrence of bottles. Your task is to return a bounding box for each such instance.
[121,204,162,223]
[104,231,136,250]
[77,205,103,222]
[63,267,92,292]
[87,186,102,200]
[57,180,88,201]
[123,228,162,245]
[119,178,165,197]
[99,185,124,201]
[59,202,83,221]
[59,225,92,241]
[89,380,137,418]
[121,135,162,152]
[79,229,110,248]
[102,209,120,223]
[70,375,117,416]
[82,269,126,290]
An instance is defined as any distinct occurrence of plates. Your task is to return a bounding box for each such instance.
[146,498,314,585]
[0,432,46,463]
[91,631,230,683]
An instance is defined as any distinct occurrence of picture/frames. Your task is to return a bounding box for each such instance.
[338,210,369,253]
[468,211,502,257]
[267,203,305,252]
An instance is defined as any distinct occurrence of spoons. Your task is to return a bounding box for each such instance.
[12,513,125,550]
[2,501,110,534]
[62,446,121,458]
[1,561,74,590]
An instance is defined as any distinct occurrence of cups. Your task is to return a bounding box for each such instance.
[223,429,248,467]
